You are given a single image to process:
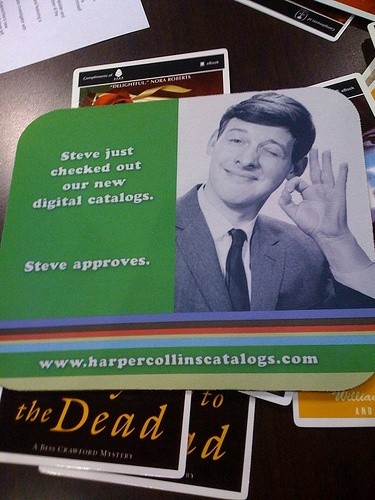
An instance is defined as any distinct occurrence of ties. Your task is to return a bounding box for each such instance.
[225,227,249,311]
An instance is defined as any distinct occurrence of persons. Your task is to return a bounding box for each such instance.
[175,92,374,312]
